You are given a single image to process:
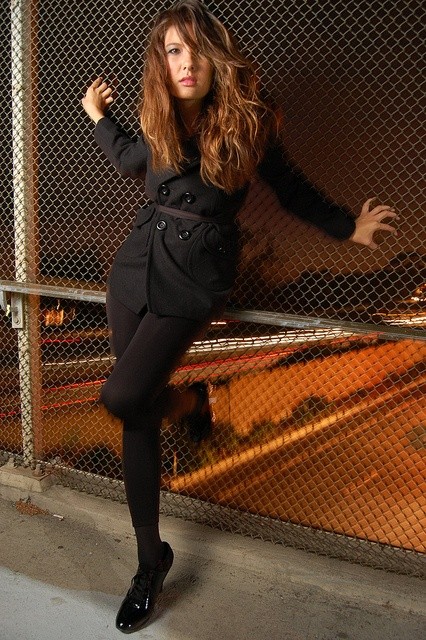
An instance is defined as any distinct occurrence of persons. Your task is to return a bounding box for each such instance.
[78,0,399,634]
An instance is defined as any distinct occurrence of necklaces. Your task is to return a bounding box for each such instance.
[177,106,203,135]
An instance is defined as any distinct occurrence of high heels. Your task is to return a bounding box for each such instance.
[114,541,175,633]
[179,381,212,443]
